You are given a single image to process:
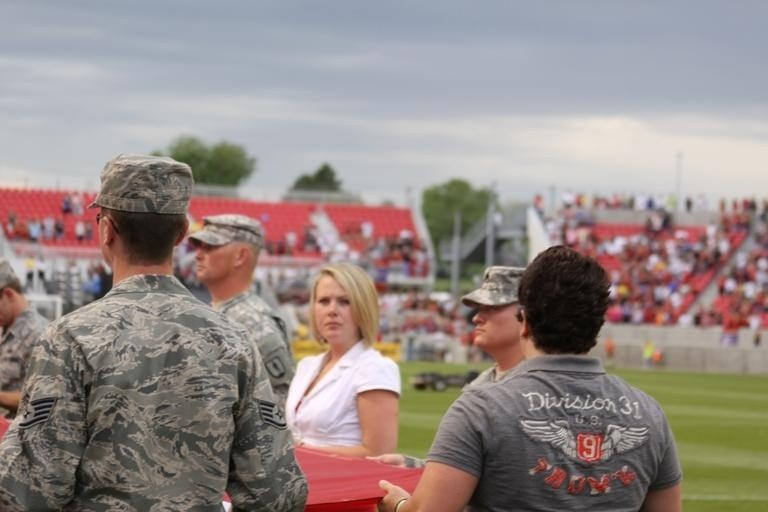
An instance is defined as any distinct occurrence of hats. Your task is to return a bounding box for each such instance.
[86,153,193,215]
[187,214,264,249]
[0,257,16,290]
[461,266,527,307]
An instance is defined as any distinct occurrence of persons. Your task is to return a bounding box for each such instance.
[24,254,48,291]
[284,262,402,464]
[83,259,113,304]
[367,264,531,468]
[380,245,682,510]
[379,288,472,361]
[532,194,768,345]
[1,259,51,411]
[7,193,93,243]
[185,214,293,400]
[1,153,309,511]
[266,222,419,262]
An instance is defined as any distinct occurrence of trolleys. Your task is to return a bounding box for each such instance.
[408,368,480,392]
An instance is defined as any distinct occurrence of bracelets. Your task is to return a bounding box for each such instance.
[393,499,407,508]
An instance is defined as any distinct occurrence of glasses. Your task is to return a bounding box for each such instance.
[97,212,120,235]
